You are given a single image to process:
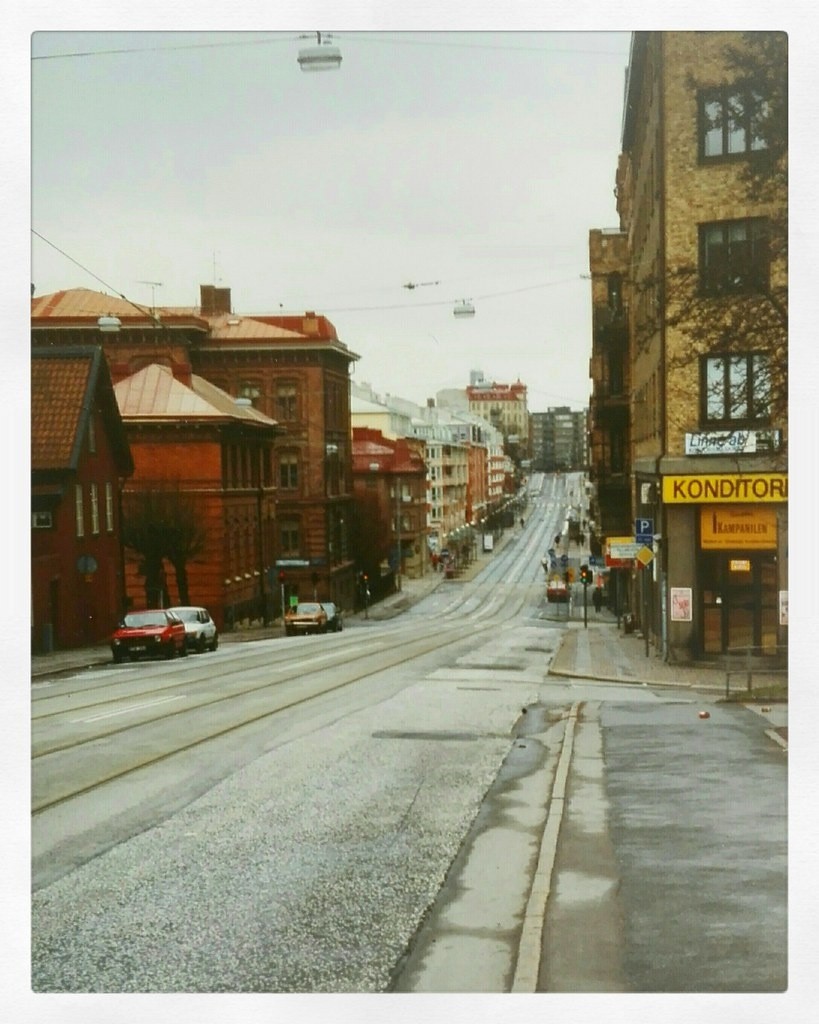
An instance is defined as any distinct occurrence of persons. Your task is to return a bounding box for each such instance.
[555,536,560,547]
[593,587,603,614]
[541,556,548,572]
[520,518,524,527]
[575,532,584,546]
[583,520,586,529]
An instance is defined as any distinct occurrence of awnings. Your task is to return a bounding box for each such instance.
[355,535,390,563]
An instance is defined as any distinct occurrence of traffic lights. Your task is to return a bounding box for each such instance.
[580,565,593,585]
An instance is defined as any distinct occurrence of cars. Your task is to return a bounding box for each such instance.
[112,609,187,658]
[315,598,344,628]
[547,581,568,603]
[170,605,218,653]
[284,601,327,633]
